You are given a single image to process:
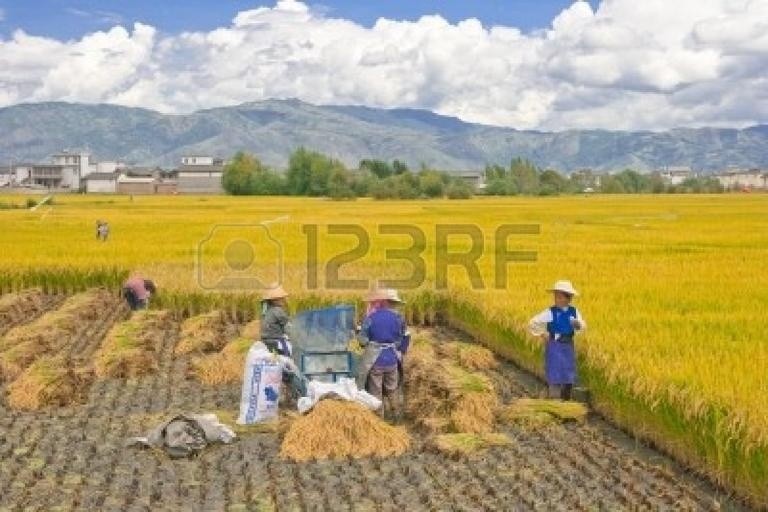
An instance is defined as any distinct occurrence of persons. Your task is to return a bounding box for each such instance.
[529,281,587,399]
[97,220,108,240]
[259,282,308,397]
[121,276,155,312]
[385,288,410,383]
[356,286,402,421]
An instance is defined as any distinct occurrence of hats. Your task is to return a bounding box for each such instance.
[263,283,289,300]
[545,280,576,296]
[362,288,402,303]
